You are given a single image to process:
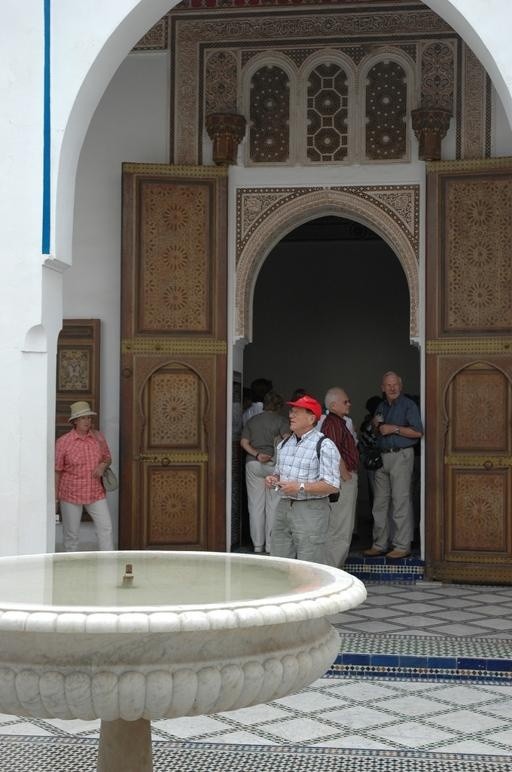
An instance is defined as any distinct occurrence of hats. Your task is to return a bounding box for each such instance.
[67,402,98,422]
[287,396,322,422]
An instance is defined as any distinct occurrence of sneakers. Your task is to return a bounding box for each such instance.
[386,550,413,558]
[364,548,382,556]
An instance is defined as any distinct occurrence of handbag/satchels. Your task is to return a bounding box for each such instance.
[101,466,118,492]
[356,441,382,471]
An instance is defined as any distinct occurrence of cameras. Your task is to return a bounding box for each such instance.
[271,481,285,492]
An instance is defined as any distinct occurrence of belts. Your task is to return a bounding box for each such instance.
[377,448,401,452]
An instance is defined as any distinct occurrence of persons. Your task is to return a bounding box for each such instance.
[56,399,118,554]
[242,368,424,573]
